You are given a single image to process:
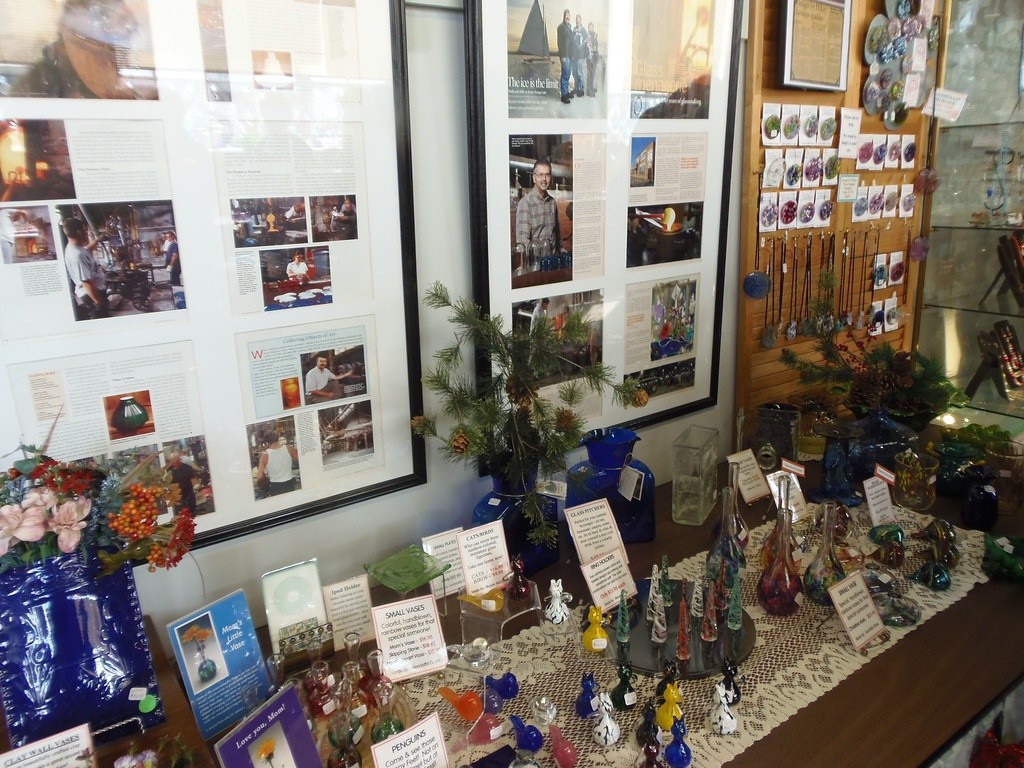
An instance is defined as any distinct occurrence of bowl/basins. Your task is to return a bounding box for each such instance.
[111,396,149,430]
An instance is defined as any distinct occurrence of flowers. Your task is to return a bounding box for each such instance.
[773,266,958,415]
[0,401,197,572]
[410,280,649,548]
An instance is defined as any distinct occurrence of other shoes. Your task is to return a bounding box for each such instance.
[561,86,597,104]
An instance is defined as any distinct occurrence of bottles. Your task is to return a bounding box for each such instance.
[239,632,404,768]
[960,464,1001,528]
[757,508,804,616]
[705,487,747,588]
[760,474,802,573]
[803,501,847,606]
[806,424,866,507]
[719,462,749,550]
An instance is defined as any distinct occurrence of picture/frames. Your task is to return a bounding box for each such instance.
[776,0,852,93]
[0,1,427,569]
[461,0,744,478]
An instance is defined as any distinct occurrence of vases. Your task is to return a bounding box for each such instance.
[0,539,168,749]
[471,454,559,579]
[842,391,971,434]
[564,429,657,545]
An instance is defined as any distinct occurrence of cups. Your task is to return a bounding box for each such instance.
[892,452,940,511]
[984,440,1024,517]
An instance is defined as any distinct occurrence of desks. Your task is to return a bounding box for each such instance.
[0,425,1024,768]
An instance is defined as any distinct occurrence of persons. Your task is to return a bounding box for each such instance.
[0,1,707,518]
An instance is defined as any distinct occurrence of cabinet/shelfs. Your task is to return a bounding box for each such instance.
[924,117,1024,420]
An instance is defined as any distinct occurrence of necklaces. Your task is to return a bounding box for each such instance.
[742,170,883,349]
[896,230,912,326]
[910,40,942,264]
